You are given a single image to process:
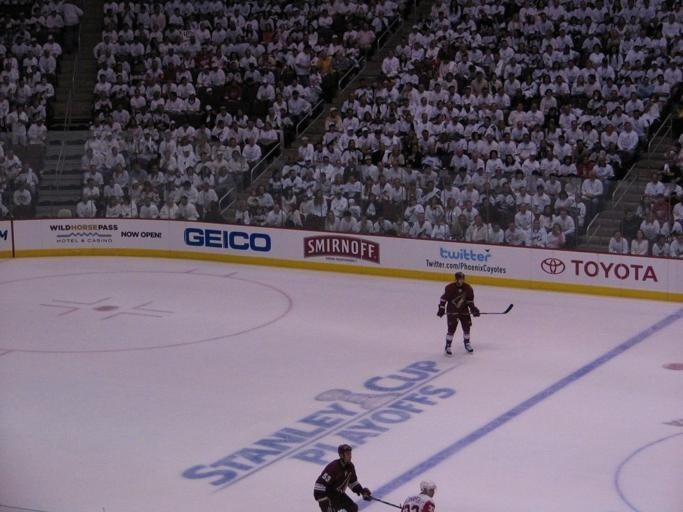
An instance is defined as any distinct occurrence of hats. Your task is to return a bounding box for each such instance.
[329,122,334,125]
[330,107,337,112]
[347,127,353,131]
[362,127,368,132]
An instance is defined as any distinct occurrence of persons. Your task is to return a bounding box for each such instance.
[314,444,372,512]
[0,0,682,258]
[401,480,437,512]
[437,273,481,354]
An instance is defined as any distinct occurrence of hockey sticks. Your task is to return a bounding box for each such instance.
[444,304,514,315]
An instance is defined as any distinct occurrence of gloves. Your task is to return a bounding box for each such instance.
[321,500,331,512]
[362,488,372,500]
[470,307,480,317]
[437,308,445,317]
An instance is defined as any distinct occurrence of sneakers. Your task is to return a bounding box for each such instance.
[465,345,473,352]
[445,346,452,354]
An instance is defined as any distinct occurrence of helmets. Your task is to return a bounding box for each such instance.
[455,272,465,279]
[420,481,436,491]
[338,444,352,456]
